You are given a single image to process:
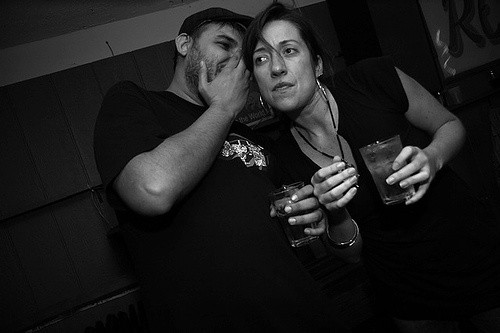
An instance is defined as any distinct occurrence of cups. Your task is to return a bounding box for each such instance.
[359,134,416,205]
[269,181,319,248]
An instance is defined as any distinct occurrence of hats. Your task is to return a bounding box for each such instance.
[177,7,254,34]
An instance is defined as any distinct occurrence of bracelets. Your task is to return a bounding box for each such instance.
[326,219,359,248]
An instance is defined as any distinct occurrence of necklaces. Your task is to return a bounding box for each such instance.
[293,78,361,188]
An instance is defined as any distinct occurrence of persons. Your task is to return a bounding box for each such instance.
[242,1,500,333]
[93,7,326,333]
